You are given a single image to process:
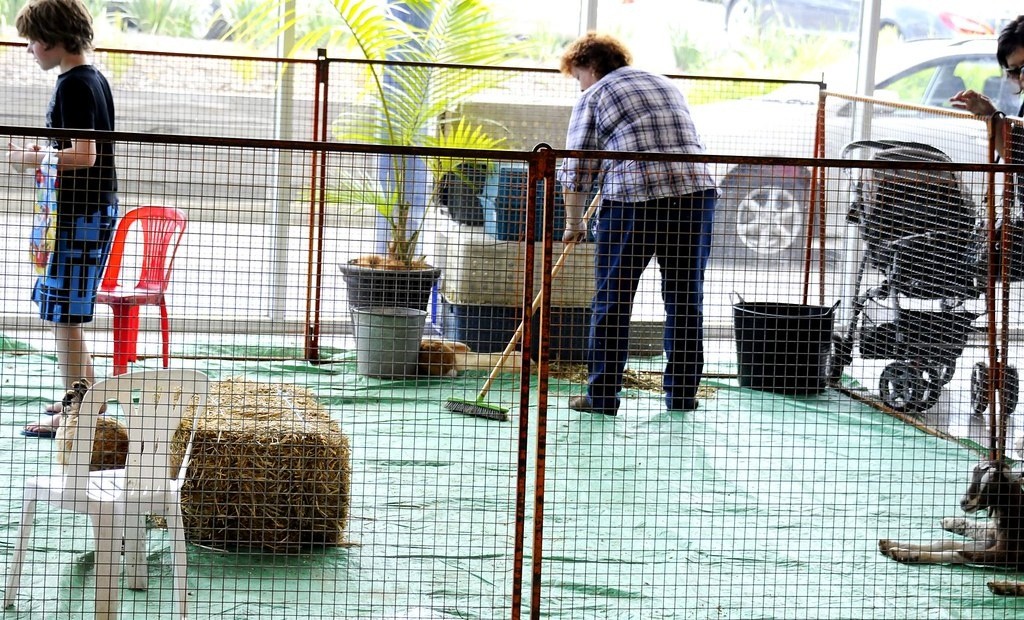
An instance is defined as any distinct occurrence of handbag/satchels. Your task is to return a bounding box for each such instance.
[964,220,1024,282]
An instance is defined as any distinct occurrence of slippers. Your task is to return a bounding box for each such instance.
[20,424,57,438]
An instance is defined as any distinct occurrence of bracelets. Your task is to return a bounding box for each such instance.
[566,219,583,225]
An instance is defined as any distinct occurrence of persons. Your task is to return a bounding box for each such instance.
[949,14,1024,216]
[7,0,121,437]
[555,32,722,416]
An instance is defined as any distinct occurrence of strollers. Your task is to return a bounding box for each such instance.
[825,138,1020,422]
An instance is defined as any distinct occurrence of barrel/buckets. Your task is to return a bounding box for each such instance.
[350,305,427,381]
[728,292,841,393]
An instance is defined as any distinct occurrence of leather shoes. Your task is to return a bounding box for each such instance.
[568,394,617,416]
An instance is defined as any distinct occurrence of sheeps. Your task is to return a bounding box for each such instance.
[877,460,1024,595]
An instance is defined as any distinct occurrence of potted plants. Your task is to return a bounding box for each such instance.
[221,0,517,349]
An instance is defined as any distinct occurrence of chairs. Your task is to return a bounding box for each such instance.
[5,370,211,620]
[93,206,186,375]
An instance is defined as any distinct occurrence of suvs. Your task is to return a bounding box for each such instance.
[675,40,1024,266]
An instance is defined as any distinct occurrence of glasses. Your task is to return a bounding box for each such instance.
[1006,65,1024,80]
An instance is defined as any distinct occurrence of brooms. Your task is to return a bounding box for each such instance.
[445,186,607,421]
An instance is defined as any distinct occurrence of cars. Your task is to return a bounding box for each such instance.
[721,0,1014,53]
